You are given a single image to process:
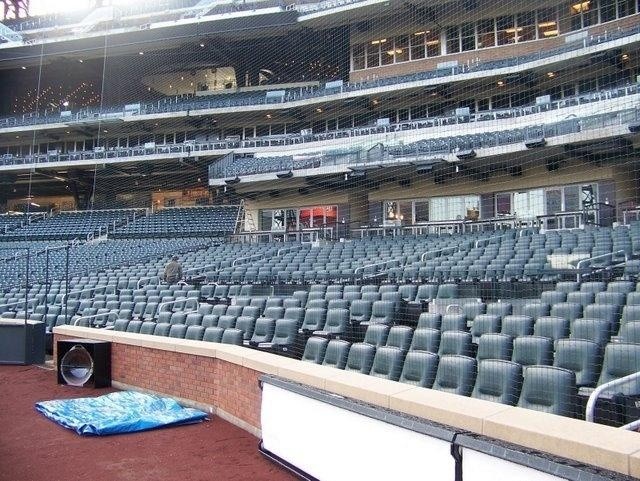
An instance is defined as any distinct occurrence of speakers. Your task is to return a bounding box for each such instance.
[57,337,112,389]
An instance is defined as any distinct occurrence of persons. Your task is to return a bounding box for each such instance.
[163,253,184,284]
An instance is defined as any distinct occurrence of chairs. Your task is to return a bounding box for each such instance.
[0,91,241,166]
[1,205,242,241]
[212,46,639,176]
[0,221,640,415]
